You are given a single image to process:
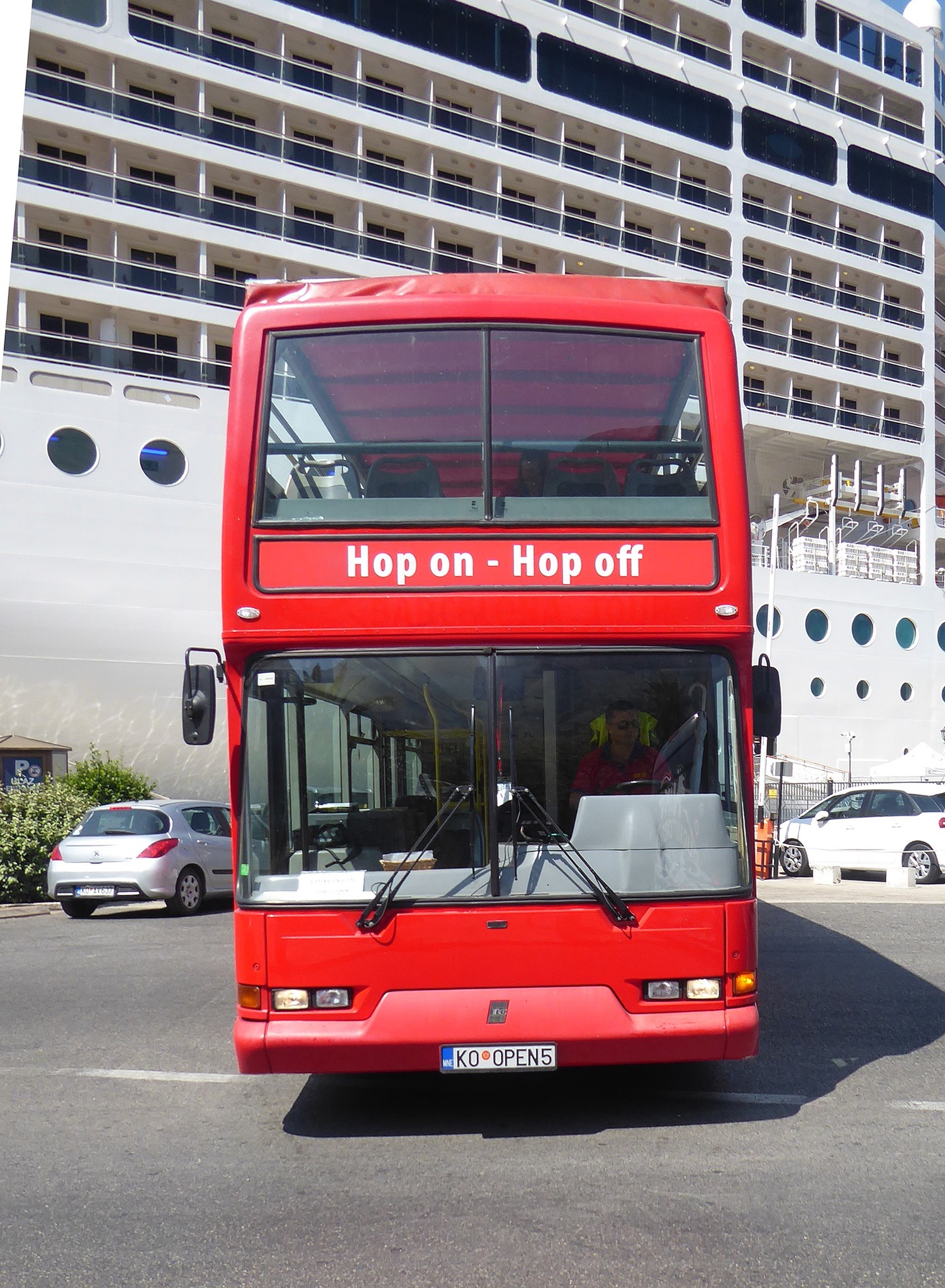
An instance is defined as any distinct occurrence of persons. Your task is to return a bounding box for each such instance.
[569,698,676,811]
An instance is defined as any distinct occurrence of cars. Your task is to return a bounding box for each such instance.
[45,800,235,920]
[774,783,945,886]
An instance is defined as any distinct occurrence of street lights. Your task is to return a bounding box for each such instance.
[840,733,856,788]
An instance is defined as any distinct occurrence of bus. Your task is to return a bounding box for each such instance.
[177,270,787,1084]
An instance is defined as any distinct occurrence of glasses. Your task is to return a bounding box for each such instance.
[609,719,639,730]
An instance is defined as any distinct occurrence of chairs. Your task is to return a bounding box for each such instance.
[883,797,899,815]
[624,458,702,497]
[281,459,362,498]
[103,813,125,834]
[588,708,658,748]
[364,456,445,497]
[138,816,160,832]
[542,457,622,497]
[190,812,213,836]
[347,806,420,855]
[392,795,440,855]
